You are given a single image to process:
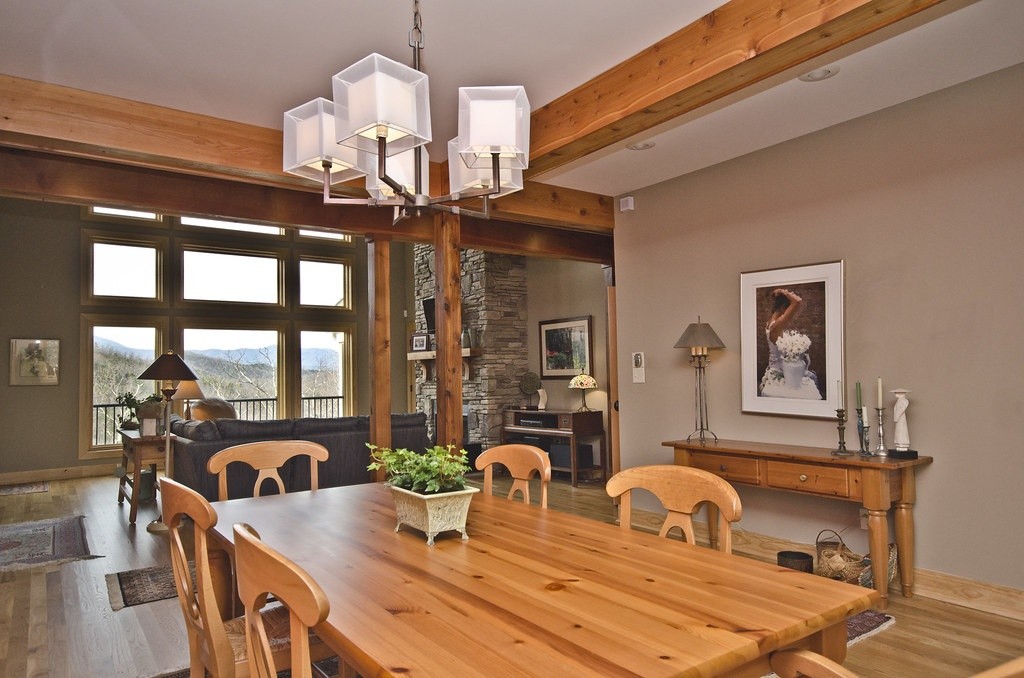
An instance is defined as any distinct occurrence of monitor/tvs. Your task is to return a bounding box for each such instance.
[422,298,435,334]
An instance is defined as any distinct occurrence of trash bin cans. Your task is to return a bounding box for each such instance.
[777,551,813,575]
[133,469,154,505]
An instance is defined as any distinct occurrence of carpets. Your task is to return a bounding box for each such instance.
[105,559,198,612]
[0,481,48,494]
[0,515,101,572]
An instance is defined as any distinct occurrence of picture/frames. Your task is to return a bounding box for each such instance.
[740,260,847,421]
[410,333,429,353]
[8,338,61,387]
[538,315,594,380]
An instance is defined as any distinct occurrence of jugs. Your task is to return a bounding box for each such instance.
[114,467,153,501]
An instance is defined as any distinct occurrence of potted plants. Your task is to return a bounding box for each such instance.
[115,391,166,435]
[365,443,480,547]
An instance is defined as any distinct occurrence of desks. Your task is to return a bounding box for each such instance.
[201,477,877,678]
[662,438,933,613]
[115,427,178,525]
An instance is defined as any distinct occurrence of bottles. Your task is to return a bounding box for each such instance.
[460,329,470,347]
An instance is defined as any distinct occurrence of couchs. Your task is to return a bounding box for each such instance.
[161,410,429,503]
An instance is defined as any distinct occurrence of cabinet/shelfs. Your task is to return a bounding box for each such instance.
[499,409,606,488]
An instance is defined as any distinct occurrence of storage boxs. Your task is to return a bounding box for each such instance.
[549,443,594,469]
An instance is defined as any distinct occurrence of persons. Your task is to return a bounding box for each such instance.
[765,288,818,385]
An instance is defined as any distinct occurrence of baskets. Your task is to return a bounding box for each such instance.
[818,551,864,581]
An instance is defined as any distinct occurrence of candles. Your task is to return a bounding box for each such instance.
[862,405,869,427]
[877,376,883,408]
[855,379,862,410]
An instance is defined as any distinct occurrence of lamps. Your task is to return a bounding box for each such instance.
[568,366,598,411]
[673,315,726,445]
[138,348,205,533]
[283,0,531,226]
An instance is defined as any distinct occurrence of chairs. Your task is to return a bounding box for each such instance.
[152,440,1024,678]
